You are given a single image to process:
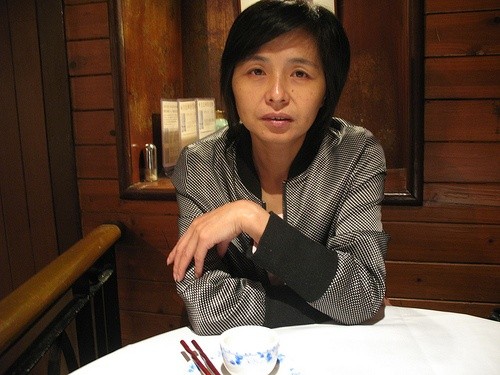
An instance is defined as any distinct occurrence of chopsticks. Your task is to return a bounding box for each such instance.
[180,340,221,375]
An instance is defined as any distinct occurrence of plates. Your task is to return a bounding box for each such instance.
[180,349,302,375]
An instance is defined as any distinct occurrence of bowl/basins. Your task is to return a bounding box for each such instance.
[220,325,279,375]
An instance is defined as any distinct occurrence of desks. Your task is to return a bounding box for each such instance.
[66,305,500,375]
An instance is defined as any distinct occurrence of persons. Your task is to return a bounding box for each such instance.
[166,0,391,335]
